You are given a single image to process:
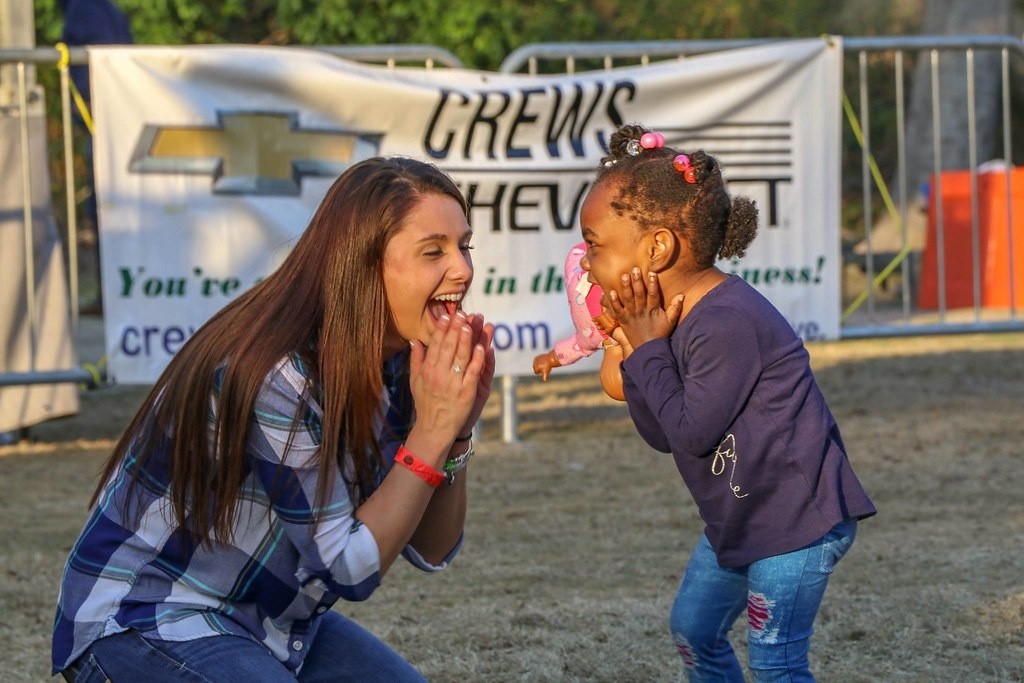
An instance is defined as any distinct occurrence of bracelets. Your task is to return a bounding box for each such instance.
[453,431,474,443]
[441,435,476,487]
[392,443,448,488]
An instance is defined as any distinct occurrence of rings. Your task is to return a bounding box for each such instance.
[451,362,465,378]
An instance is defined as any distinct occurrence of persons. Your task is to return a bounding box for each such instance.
[531,243,631,402]
[48,155,496,682]
[578,122,880,682]
